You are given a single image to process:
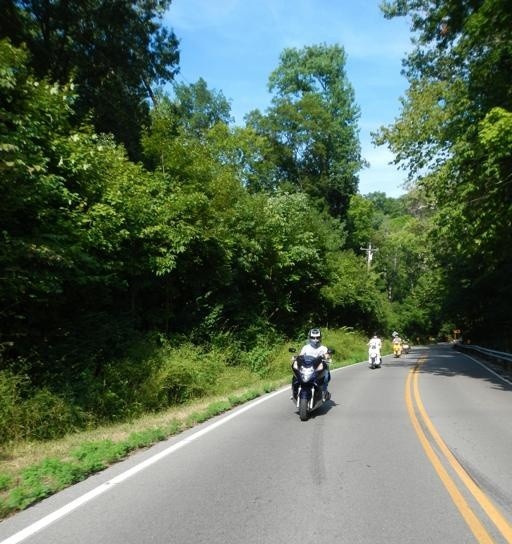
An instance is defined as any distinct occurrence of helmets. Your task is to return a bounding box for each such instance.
[309,329,323,348]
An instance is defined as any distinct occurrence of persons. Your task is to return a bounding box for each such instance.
[402,336,411,351]
[392,335,401,344]
[290,327,331,405]
[366,333,382,363]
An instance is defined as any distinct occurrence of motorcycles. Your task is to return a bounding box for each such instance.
[402,340,411,353]
[391,341,403,357]
[367,343,382,369]
[286,346,336,421]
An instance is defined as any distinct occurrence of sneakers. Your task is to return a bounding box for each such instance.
[322,392,326,402]
